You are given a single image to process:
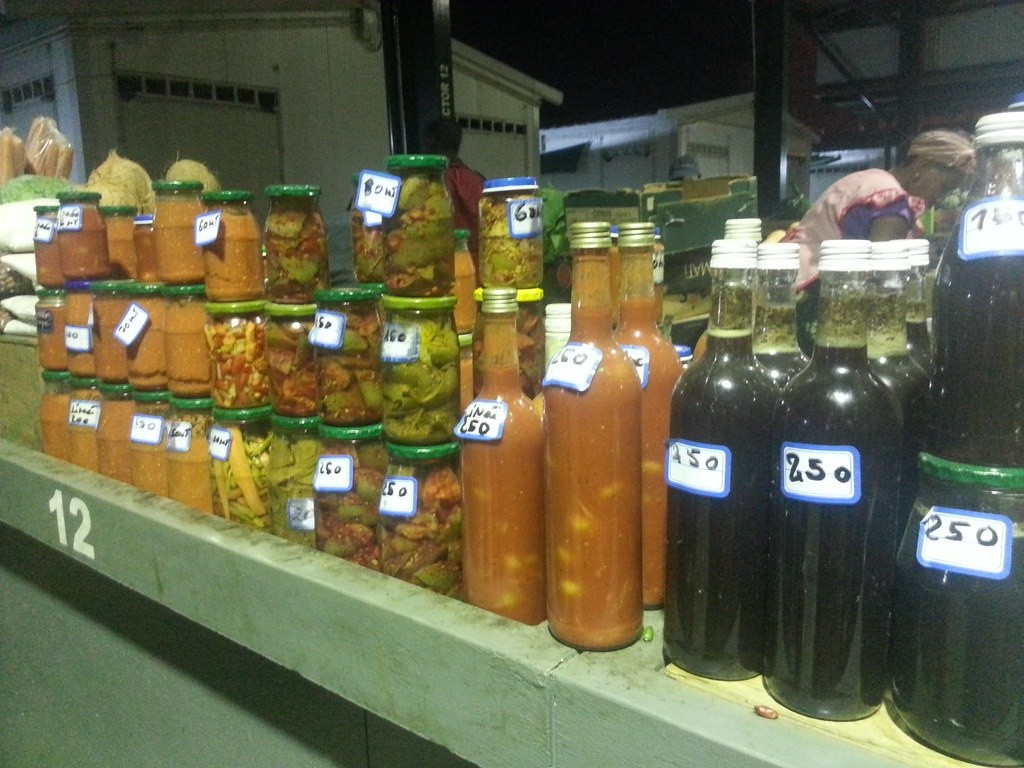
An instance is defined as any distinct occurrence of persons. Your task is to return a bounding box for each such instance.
[780,126,974,360]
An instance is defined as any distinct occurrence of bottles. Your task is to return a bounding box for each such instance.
[461,93,1024,768]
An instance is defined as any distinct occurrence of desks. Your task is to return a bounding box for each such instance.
[2,438,983,768]
